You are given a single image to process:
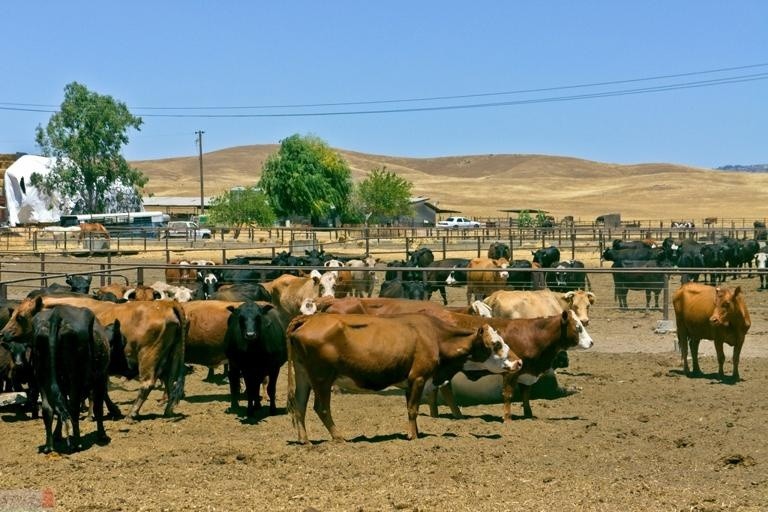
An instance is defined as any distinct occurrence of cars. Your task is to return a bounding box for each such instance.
[438,216,481,229]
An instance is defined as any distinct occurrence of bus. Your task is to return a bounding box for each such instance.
[60,211,164,237]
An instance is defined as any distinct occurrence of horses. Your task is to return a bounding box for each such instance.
[41,222,110,248]
[671,222,695,238]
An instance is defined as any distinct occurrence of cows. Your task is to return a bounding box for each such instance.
[599,237,768,313]
[0,241,596,455]
[671,281,751,380]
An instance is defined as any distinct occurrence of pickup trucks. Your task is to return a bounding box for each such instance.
[160,221,211,239]
